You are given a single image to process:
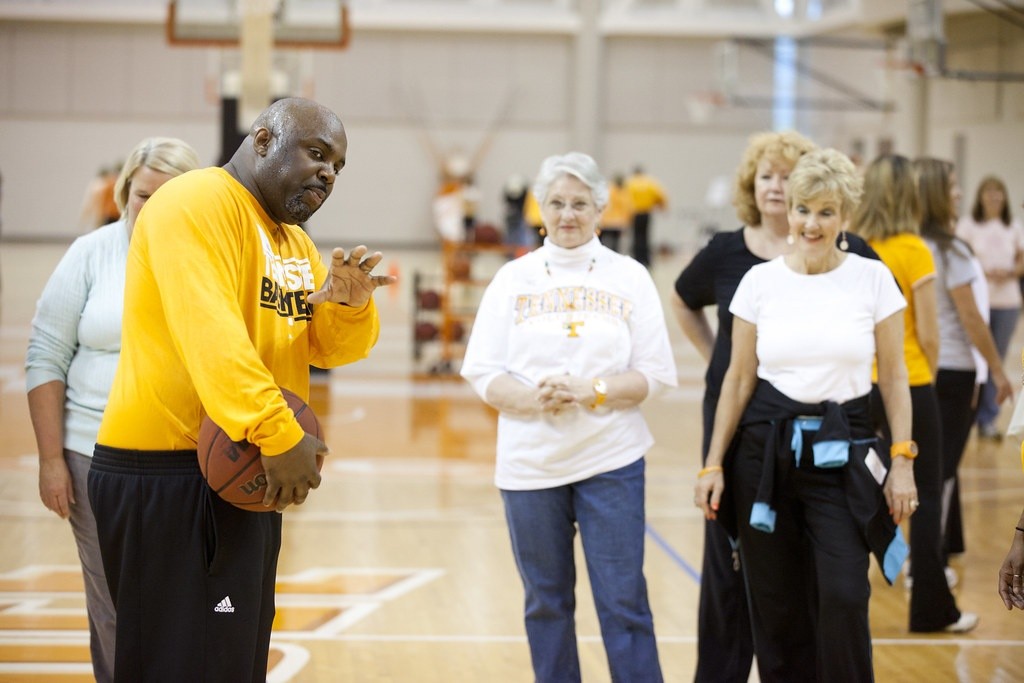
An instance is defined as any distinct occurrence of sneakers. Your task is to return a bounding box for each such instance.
[939,611,980,633]
[905,564,959,590]
[977,421,1004,441]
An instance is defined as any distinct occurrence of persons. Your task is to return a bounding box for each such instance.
[902,158,1012,598]
[521,158,734,269]
[840,153,980,635]
[77,160,129,232]
[21,136,207,682]
[670,123,905,683]
[85,96,399,683]
[458,148,680,683]
[690,145,919,683]
[954,171,1024,443]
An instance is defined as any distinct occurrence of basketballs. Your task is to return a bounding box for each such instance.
[450,260,469,280]
[446,321,463,340]
[421,291,439,310]
[475,225,500,246]
[196,386,324,513]
[416,323,437,341]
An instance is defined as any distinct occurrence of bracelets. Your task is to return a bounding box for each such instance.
[1015,527,1024,532]
[696,465,723,477]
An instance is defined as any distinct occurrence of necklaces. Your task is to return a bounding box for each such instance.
[541,246,601,339]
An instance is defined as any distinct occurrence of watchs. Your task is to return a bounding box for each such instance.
[586,377,608,413]
[889,440,919,460]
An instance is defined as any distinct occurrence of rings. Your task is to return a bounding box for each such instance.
[1013,573,1022,577]
[909,501,919,506]
